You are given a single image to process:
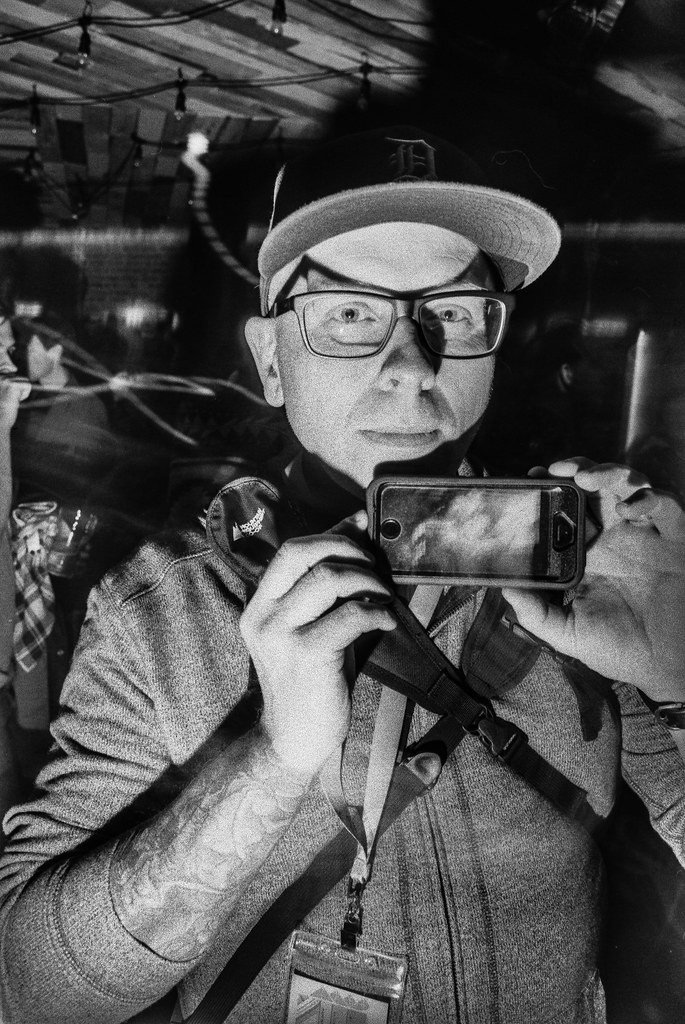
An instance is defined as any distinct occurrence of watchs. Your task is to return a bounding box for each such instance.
[636,687,685,732]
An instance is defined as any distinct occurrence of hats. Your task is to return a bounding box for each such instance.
[258,119,561,317]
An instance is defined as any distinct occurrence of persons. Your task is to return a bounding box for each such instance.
[0,124,685,1024]
[0,303,117,817]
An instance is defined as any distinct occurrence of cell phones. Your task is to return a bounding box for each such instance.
[365,478,585,589]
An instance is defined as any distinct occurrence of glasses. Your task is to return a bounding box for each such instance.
[262,289,516,360]
